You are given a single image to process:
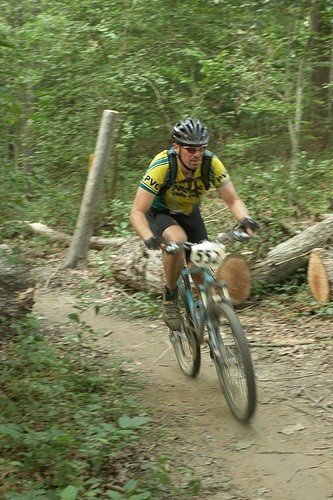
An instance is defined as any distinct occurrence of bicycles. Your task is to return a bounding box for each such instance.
[152,224,259,422]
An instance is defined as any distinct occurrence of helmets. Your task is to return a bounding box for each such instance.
[169,118,210,146]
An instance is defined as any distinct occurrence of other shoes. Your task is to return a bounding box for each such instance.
[162,290,182,331]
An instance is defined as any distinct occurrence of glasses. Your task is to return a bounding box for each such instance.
[182,145,206,154]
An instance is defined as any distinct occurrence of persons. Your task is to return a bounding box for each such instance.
[130,117,261,331]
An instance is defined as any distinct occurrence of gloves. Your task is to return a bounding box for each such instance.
[238,216,261,235]
[144,235,166,251]
